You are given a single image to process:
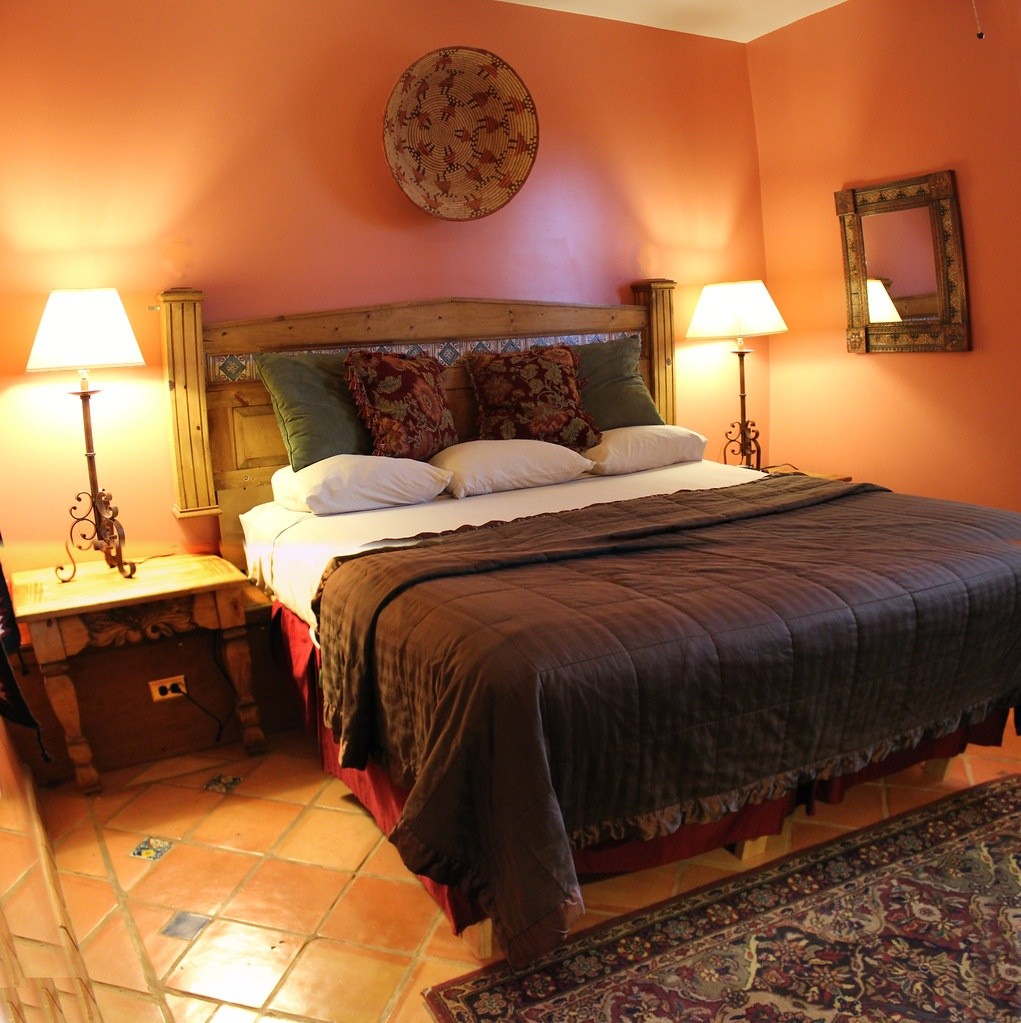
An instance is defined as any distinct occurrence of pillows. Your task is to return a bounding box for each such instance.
[271,453,452,514]
[463,343,602,456]
[578,423,710,476]
[341,346,460,460]
[568,334,669,429]
[250,348,372,472]
[426,438,597,499]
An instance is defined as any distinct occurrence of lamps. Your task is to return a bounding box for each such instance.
[685,279,789,466]
[865,277,902,323]
[21,286,146,582]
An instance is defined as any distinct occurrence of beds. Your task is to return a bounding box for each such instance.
[156,278,1021,972]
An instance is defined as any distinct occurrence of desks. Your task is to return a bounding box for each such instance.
[14,550,268,797]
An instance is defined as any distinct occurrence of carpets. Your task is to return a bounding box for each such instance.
[415,773,1020,1023]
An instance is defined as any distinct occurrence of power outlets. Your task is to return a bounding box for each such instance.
[148,675,188,703]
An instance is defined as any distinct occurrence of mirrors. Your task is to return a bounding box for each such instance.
[834,169,972,352]
